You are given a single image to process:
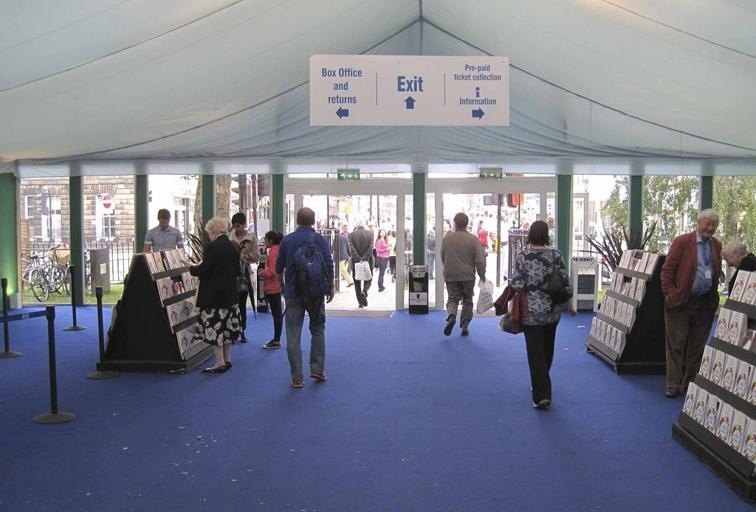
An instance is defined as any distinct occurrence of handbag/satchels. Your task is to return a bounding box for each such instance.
[477,278,494,315]
[355,260,372,281]
[549,267,573,304]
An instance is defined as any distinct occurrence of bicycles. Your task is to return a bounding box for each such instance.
[20,242,90,302]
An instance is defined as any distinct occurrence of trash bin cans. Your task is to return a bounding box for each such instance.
[90,248,110,295]
[409,265,428,314]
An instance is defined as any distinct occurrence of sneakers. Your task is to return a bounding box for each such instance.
[263,338,281,349]
[444,318,456,336]
[533,399,551,408]
[293,380,305,388]
[359,292,368,306]
[310,370,327,381]
[461,329,469,336]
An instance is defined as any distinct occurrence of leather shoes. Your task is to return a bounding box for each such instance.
[666,386,678,399]
[203,362,232,372]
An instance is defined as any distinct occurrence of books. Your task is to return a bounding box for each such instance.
[591,248,659,354]
[145,245,207,358]
[681,269,756,464]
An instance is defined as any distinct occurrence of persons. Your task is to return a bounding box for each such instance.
[440,212,487,337]
[520,222,530,230]
[274,206,335,389]
[143,208,185,252]
[329,217,484,308]
[247,223,254,233]
[181,216,244,373]
[659,208,723,398]
[508,220,579,410]
[721,239,756,299]
[258,230,285,349]
[229,212,259,344]
[508,220,518,229]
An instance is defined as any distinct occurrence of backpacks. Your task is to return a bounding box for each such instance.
[293,232,332,303]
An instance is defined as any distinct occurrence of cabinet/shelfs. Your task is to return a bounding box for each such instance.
[570,256,599,314]
[97,249,214,373]
[586,250,667,377]
[670,268,756,504]
